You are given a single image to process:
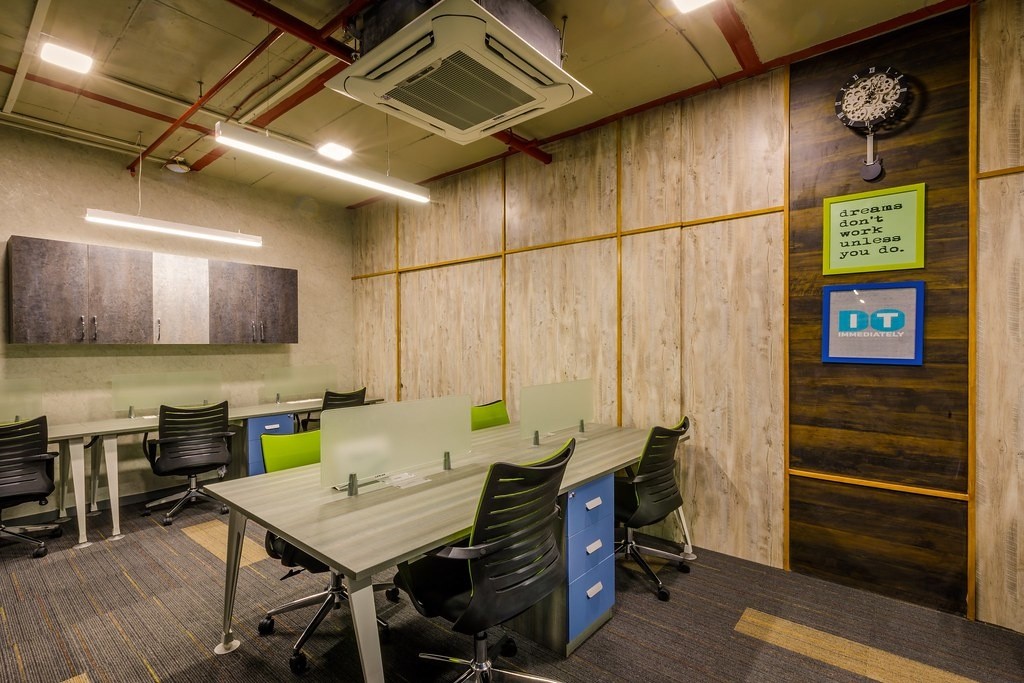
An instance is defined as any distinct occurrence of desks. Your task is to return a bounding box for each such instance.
[48,399,322,543]
[202,419,652,683]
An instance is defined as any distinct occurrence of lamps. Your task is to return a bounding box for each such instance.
[84,131,263,248]
[213,121,431,204]
[166,160,191,174]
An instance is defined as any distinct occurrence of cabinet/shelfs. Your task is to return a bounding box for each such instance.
[6,235,299,345]
[503,473,616,659]
[246,414,294,475]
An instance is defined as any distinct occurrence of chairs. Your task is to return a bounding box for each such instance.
[142,400,236,526]
[471,399,511,431]
[615,415,697,602]
[0,415,59,558]
[302,386,366,431]
[393,437,576,683]
[260,431,400,669]
